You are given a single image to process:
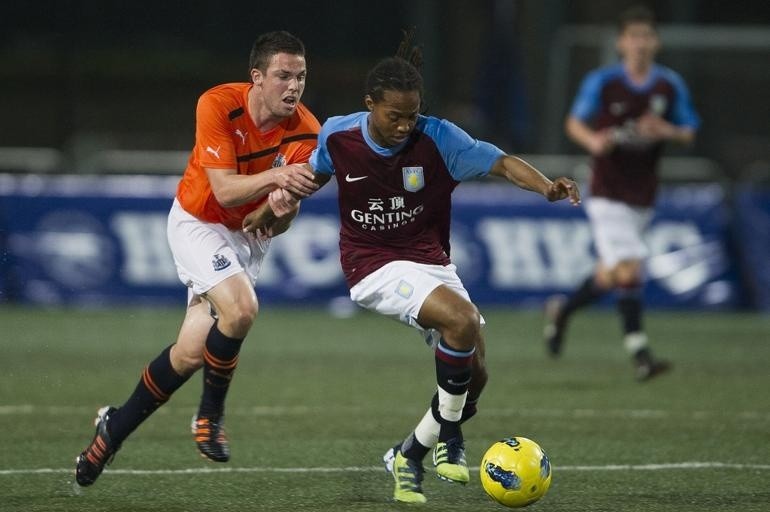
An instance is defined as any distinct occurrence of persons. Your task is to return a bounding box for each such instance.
[539,8,702,382]
[77,27,324,489]
[242,31,583,504]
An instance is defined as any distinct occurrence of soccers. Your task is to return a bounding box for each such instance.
[480,437,553,506]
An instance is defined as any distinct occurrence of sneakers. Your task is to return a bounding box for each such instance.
[432,437,470,485]
[191,410,230,462]
[382,443,428,505]
[542,294,572,356]
[75,405,117,488]
[633,349,667,380]
